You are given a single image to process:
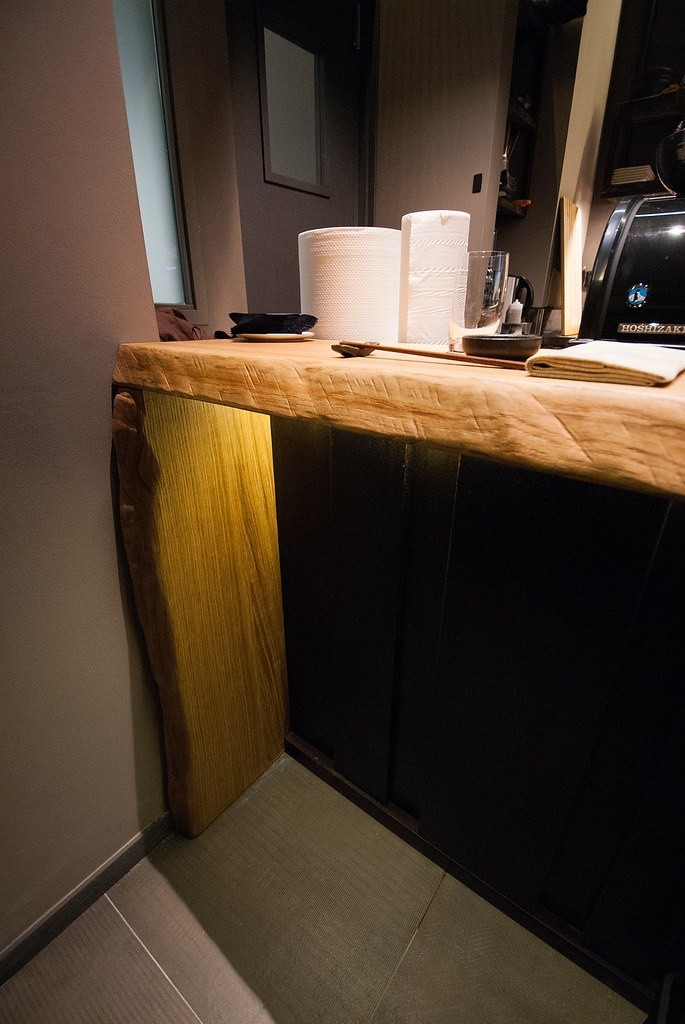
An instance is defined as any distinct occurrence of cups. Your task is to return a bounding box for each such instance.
[449,250,509,351]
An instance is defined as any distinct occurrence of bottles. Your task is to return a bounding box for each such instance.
[508,299,524,324]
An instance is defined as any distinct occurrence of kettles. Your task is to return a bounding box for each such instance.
[501,273,535,336]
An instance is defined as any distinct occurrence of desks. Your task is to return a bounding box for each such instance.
[110,336,684,842]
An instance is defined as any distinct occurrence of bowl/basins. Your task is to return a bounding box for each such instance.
[462,335,542,362]
[227,312,318,334]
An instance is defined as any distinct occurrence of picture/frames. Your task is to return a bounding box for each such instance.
[259,20,334,199]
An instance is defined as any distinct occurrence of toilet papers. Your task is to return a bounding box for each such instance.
[399,211,469,352]
[300,226,401,341]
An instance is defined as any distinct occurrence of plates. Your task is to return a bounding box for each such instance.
[236,331,314,341]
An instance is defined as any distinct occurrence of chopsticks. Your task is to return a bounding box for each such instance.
[339,341,526,370]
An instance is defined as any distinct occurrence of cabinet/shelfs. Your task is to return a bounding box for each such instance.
[498,7,540,217]
